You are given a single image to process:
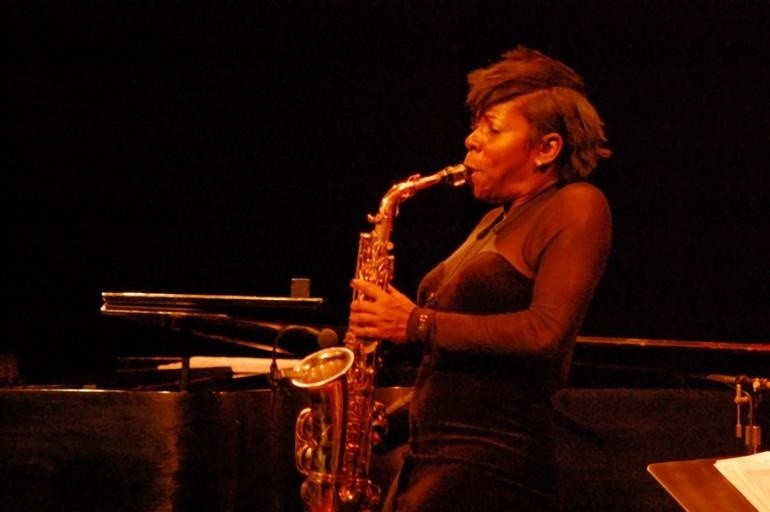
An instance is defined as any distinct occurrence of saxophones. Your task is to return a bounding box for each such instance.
[290,164,473,512]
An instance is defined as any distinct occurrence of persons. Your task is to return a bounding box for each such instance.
[346,44,614,512]
